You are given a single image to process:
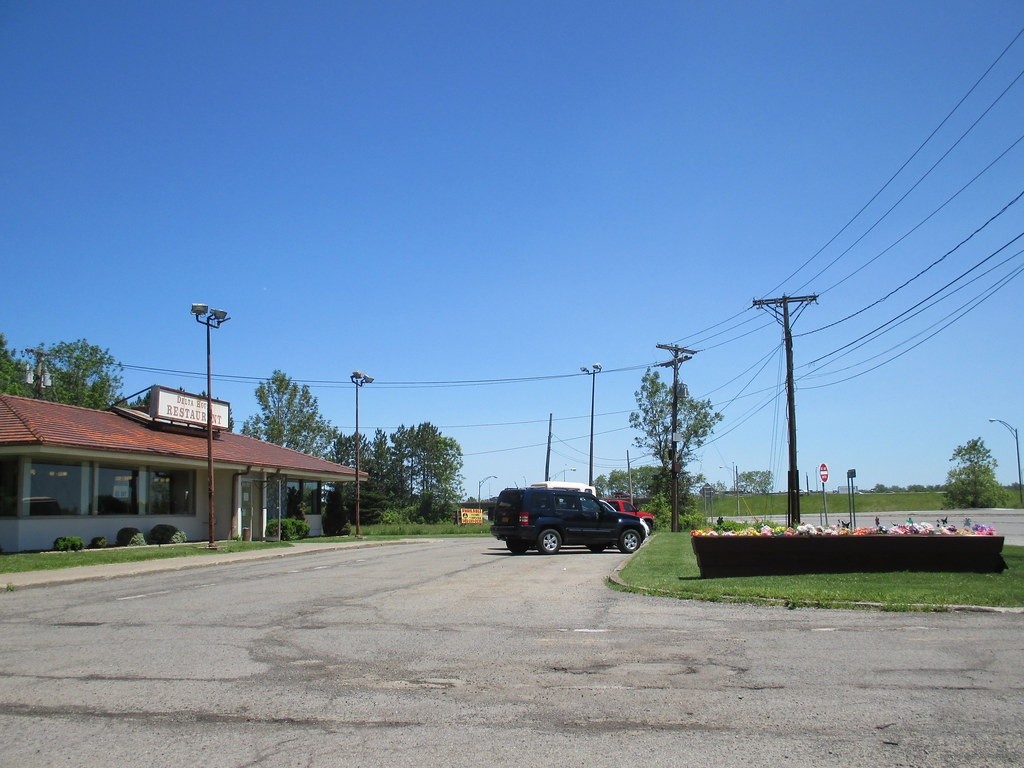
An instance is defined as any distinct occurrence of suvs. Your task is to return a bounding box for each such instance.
[489,487,649,555]
[604,499,655,536]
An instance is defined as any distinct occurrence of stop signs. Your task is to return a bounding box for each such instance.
[819,464,828,483]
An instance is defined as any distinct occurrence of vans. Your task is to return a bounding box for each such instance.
[532,482,596,502]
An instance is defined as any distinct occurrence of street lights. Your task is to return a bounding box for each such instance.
[189,302,232,552]
[989,417,1023,505]
[477,475,497,510]
[349,369,374,539]
[580,363,603,486]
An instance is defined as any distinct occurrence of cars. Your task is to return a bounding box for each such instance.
[797,488,895,494]
[558,499,650,548]
